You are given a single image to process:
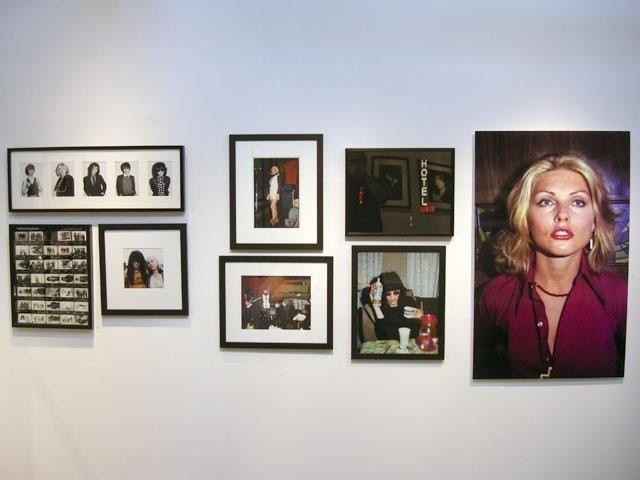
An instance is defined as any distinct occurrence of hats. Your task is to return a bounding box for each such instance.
[379,271,403,290]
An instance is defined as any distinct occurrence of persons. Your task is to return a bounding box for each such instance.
[266,165,281,224]
[370,271,422,340]
[146,255,164,288]
[83,163,106,196]
[149,162,170,196]
[262,286,270,309]
[124,251,147,288]
[116,162,136,196]
[54,163,74,196]
[22,163,42,197]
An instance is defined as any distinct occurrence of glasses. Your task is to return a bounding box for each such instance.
[386,290,401,296]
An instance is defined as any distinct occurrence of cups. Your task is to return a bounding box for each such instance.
[399,327,411,346]
[403,306,415,319]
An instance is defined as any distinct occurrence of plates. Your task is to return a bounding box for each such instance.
[418,345,437,351]
[393,346,416,354]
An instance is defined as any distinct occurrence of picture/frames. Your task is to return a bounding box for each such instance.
[345,147,454,237]
[351,245,448,359]
[219,255,334,350]
[6,147,184,211]
[229,133,323,252]
[9,224,94,331]
[99,224,189,316]
